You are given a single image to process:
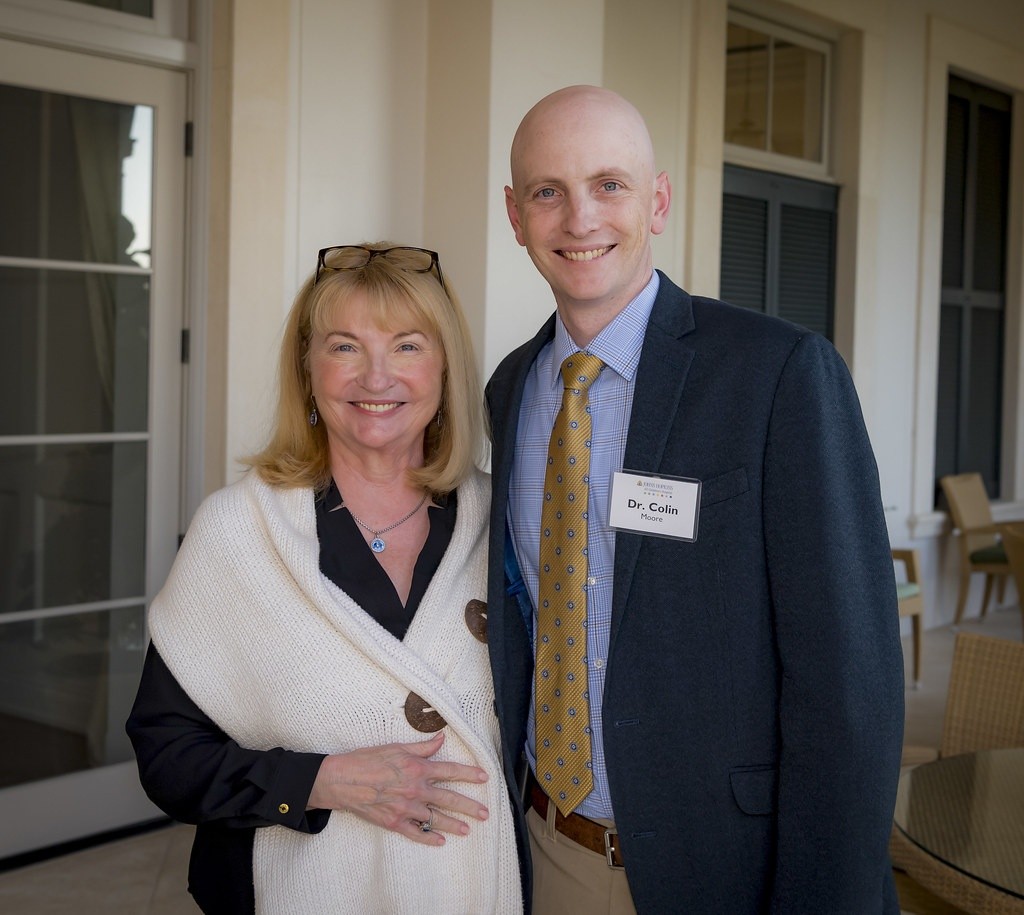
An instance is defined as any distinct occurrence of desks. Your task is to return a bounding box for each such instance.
[885,747,1024,915]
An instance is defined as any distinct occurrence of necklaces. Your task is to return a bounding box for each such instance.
[343,489,431,552]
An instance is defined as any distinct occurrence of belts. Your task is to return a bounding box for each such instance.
[526,783,621,869]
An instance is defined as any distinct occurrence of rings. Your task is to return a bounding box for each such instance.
[418,807,434,832]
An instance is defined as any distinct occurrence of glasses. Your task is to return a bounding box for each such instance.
[314,245,446,292]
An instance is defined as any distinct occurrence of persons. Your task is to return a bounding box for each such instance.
[123,240,528,915]
[482,82,906,915]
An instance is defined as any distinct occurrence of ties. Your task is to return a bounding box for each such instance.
[533,353,605,818]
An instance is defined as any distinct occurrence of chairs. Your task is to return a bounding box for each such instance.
[941,471,1024,627]
[1003,526,1024,623]
[888,547,926,690]
[888,630,1024,776]
